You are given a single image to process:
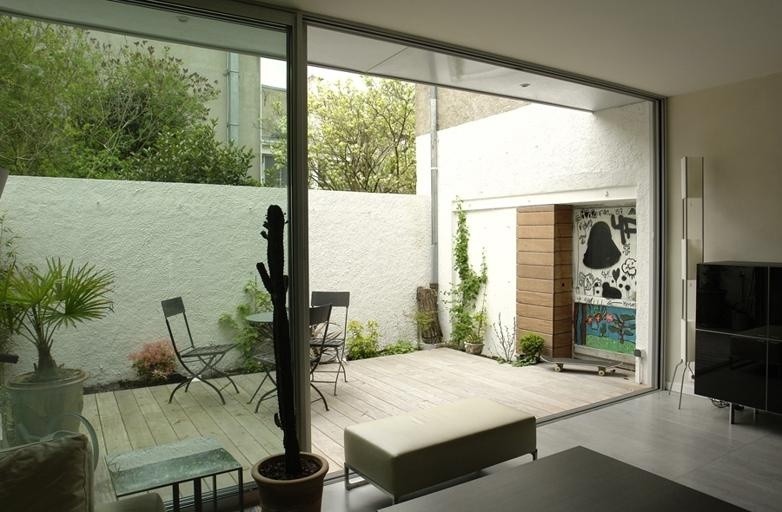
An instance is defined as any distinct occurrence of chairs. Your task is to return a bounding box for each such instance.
[309,291,350,396]
[160,297,242,406]
[254,302,333,412]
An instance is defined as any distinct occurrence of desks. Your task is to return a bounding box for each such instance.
[108,446,245,512]
[375,444,753,512]
[245,312,275,405]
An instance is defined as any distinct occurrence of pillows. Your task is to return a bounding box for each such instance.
[0,433,94,512]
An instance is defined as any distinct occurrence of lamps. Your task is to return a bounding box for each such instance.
[667,155,706,409]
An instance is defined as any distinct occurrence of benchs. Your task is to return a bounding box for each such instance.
[343,395,538,505]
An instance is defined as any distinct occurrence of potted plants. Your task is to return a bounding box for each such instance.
[463,334,485,355]
[250,203,330,512]
[0,254,117,438]
[440,195,487,351]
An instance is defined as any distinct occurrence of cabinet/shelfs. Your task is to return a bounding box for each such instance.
[696,261,782,425]
